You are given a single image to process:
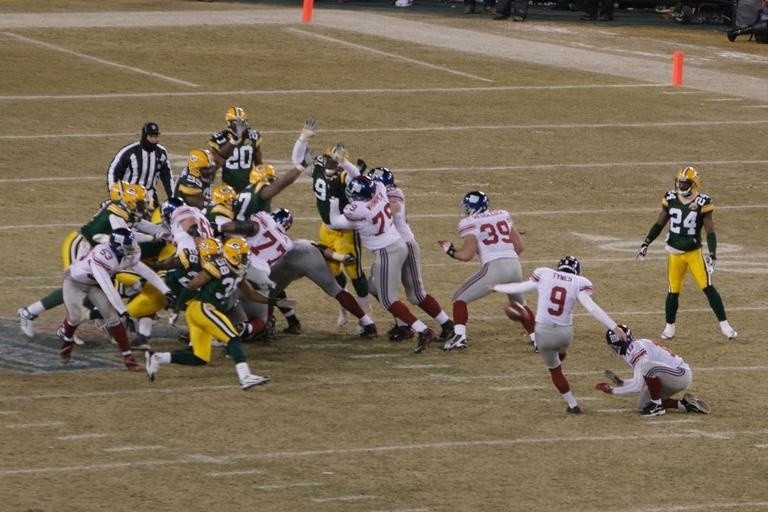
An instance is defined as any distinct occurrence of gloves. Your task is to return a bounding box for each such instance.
[230,116,248,145]
[438,240,456,258]
[595,382,613,395]
[355,159,367,176]
[635,241,648,260]
[268,297,297,309]
[709,253,716,271]
[605,370,624,387]
[119,311,130,329]
[332,142,345,165]
[164,291,177,310]
[302,146,318,168]
[298,117,319,143]
[343,252,356,266]
[168,309,179,326]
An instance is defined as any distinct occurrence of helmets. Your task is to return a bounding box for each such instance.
[174,174,204,198]
[224,106,248,135]
[459,191,489,218]
[109,227,137,257]
[557,255,581,275]
[210,183,236,220]
[110,180,129,204]
[672,168,701,199]
[249,163,279,185]
[120,184,150,222]
[199,238,222,257]
[223,236,251,277]
[606,324,633,356]
[323,144,348,180]
[160,197,186,223]
[271,208,294,234]
[344,175,376,204]
[188,148,216,183]
[368,167,397,189]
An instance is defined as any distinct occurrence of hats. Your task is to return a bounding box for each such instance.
[142,122,160,135]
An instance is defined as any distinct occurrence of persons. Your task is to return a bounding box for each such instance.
[595,324,711,417]
[438,190,539,353]
[201,184,260,242]
[327,175,435,354]
[99,179,171,242]
[208,106,264,193]
[58,228,178,373]
[117,237,224,316]
[366,166,454,342]
[105,122,174,213]
[485,256,630,415]
[635,166,738,340]
[292,117,368,334]
[16,185,149,339]
[174,107,251,200]
[152,173,214,257]
[232,190,377,338]
[145,236,296,391]
[230,145,318,333]
[229,208,293,339]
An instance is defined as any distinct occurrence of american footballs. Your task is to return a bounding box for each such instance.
[504,301,528,321]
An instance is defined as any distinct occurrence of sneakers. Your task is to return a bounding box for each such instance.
[128,332,151,350]
[56,325,85,345]
[16,307,38,339]
[359,324,377,338]
[719,320,738,339]
[236,363,270,390]
[355,306,371,336]
[144,348,171,384]
[660,322,676,339]
[678,393,711,415]
[639,398,666,416]
[529,332,539,352]
[439,333,469,352]
[60,336,73,364]
[433,319,456,343]
[336,309,349,329]
[389,327,414,342]
[387,324,413,334]
[121,350,141,371]
[284,322,302,334]
[414,327,436,354]
[565,406,581,414]
